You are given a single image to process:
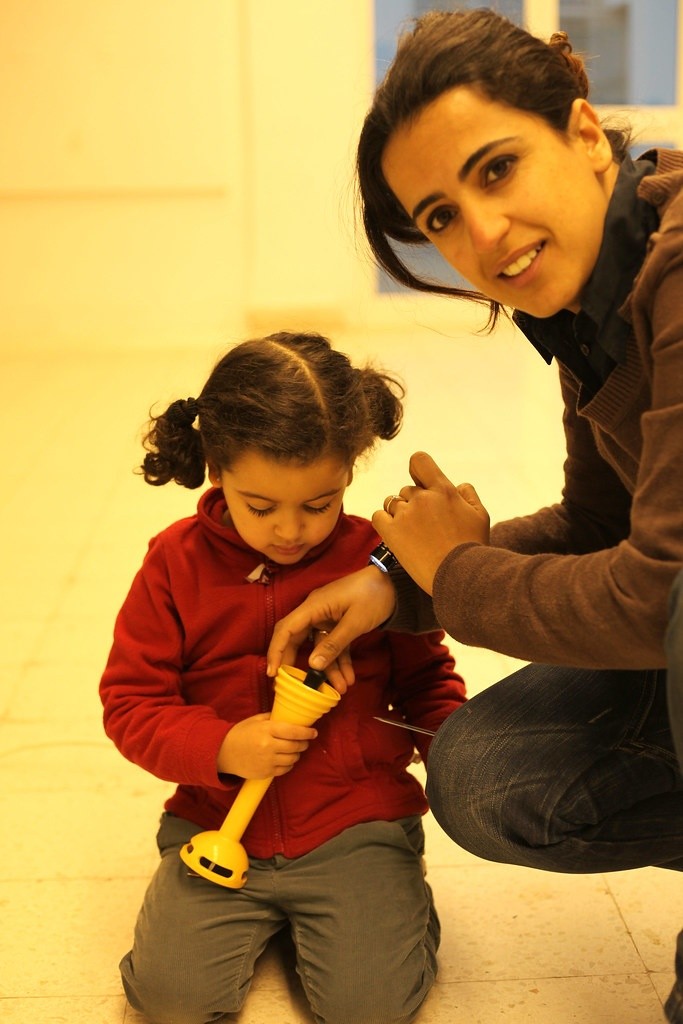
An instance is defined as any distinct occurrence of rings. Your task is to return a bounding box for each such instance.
[312,626,330,638]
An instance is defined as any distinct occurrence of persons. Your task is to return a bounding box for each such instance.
[264,5,682,1024]
[98,331,470,1024]
